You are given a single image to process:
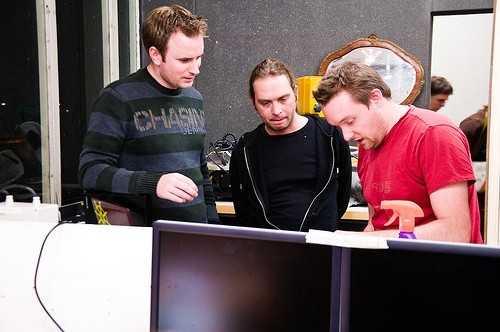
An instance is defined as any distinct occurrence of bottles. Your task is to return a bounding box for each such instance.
[6,195,14,203]
[81,189,99,224]
[32,197,42,204]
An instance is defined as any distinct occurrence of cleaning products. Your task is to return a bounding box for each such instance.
[380,200,424,240]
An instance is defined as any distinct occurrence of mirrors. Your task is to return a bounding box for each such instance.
[317,32,425,106]
[428,9,497,162]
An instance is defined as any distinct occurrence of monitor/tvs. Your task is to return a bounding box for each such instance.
[150,219,343,332]
[339,237,500,332]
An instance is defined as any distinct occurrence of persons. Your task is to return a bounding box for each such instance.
[430,76,453,113]
[229,60,353,231]
[458,106,488,242]
[77,4,223,227]
[312,60,485,246]
